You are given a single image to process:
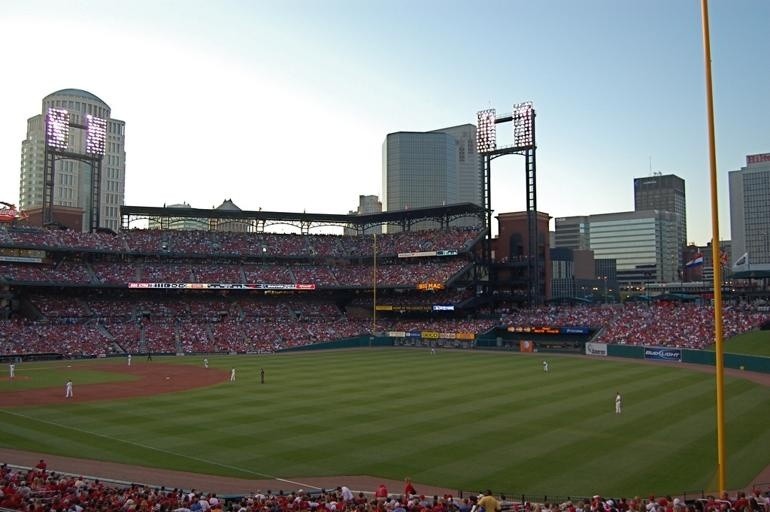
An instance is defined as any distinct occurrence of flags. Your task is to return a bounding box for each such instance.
[686,251,704,272]
[711,248,731,271]
[732,251,748,272]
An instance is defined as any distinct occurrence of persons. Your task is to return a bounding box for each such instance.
[10,363,18,379]
[1,220,507,362]
[229,367,238,383]
[259,367,266,384]
[541,359,551,374]
[127,351,132,367]
[508,286,770,350]
[0,457,770,512]
[614,390,625,415]
[203,355,210,369]
[64,379,75,399]
